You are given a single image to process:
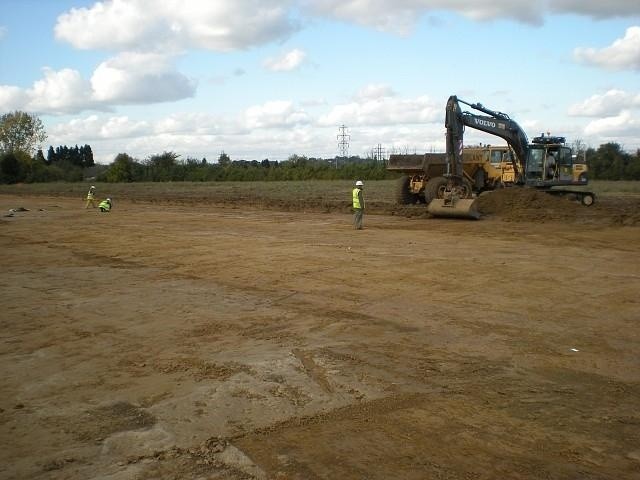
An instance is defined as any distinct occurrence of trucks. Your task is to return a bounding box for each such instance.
[384,142,523,205]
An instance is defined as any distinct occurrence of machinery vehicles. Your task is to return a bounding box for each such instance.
[426,95,598,221]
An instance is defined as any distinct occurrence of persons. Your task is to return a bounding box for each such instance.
[97,198,113,212]
[542,151,556,178]
[85,186,98,209]
[352,181,365,230]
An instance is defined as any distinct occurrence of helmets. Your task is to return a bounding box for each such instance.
[355,180,364,186]
[90,186,96,189]
[107,198,111,201]
